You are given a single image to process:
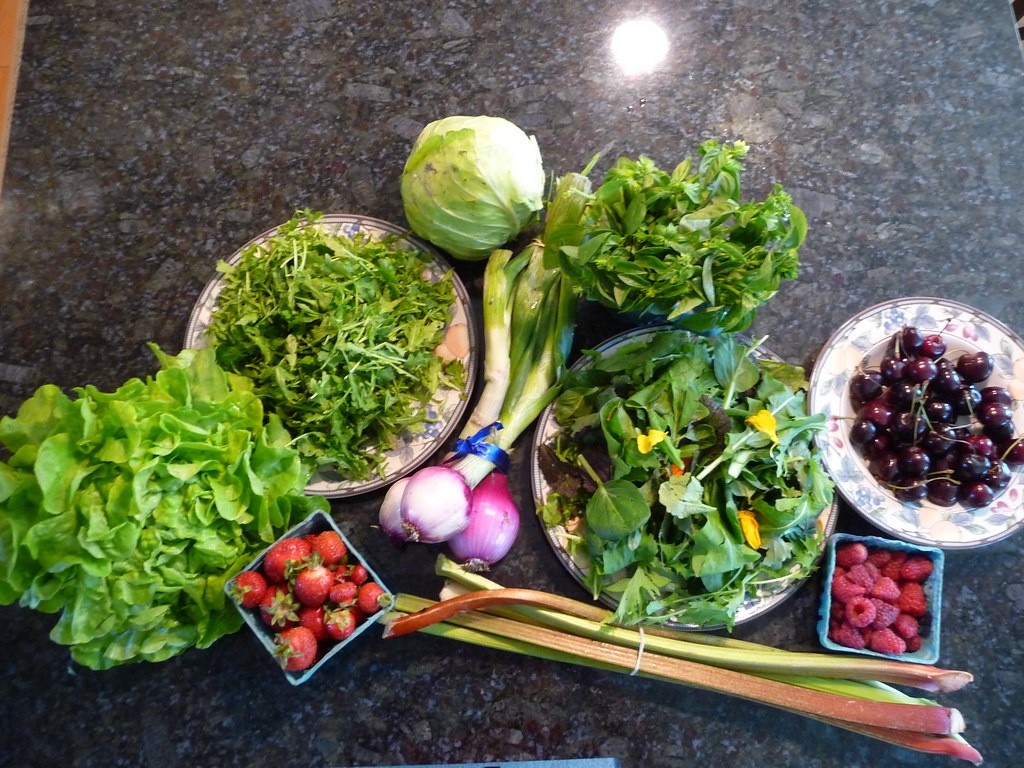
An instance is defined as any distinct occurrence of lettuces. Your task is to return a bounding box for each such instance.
[0,343,328,674]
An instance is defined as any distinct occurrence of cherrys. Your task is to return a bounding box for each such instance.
[838,315,1024,507]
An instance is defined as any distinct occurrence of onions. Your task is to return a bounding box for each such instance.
[376,463,520,572]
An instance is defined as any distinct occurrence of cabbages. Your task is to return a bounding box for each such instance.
[398,115,549,260]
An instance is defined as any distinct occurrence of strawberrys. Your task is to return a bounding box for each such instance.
[230,533,391,673]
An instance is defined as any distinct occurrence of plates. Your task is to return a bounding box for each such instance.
[805,293,1023,551]
[181,214,479,501]
[530,318,839,634]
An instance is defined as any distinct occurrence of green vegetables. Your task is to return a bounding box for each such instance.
[536,136,837,634]
[193,209,469,484]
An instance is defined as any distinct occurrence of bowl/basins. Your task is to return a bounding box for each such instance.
[814,528,946,666]
[221,508,396,686]
